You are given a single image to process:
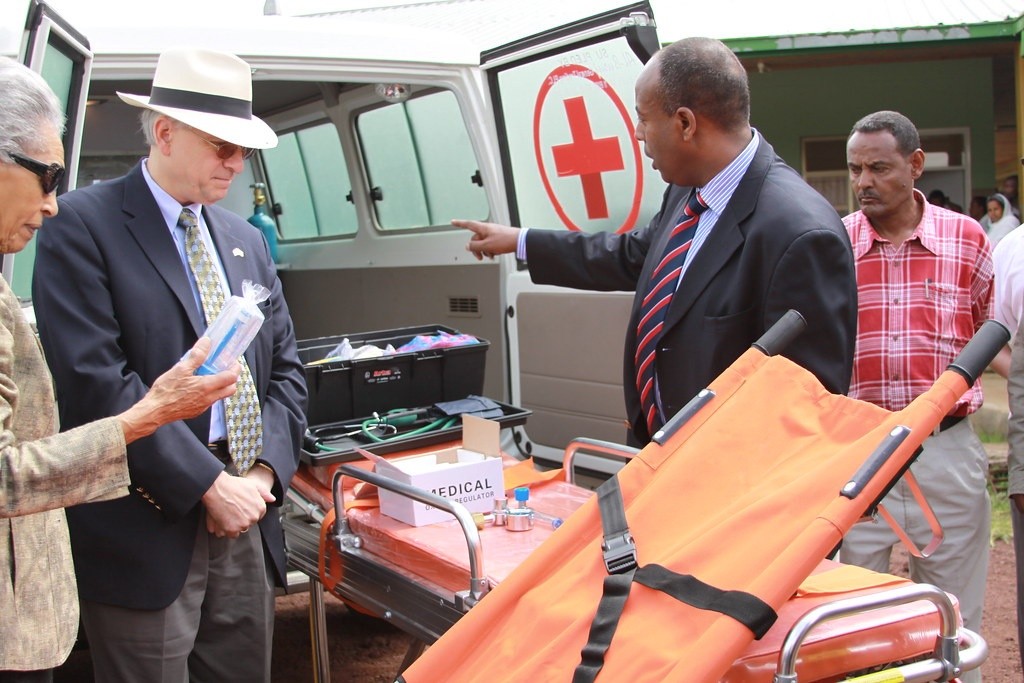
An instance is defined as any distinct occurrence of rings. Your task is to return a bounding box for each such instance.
[240,528,249,533]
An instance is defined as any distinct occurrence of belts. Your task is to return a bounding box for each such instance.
[928,416,963,437]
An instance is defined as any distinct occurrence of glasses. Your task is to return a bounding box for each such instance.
[188,129,256,160]
[6,151,64,193]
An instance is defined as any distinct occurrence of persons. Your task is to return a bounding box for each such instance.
[451,37,859,464]
[841,110,1024,683]
[0,48,309,683]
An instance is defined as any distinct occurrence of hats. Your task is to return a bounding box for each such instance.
[116,49,279,150]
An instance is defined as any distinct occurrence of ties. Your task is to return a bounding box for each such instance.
[635,191,703,437]
[178,210,262,478]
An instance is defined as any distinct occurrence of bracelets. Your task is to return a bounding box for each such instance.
[257,462,275,474]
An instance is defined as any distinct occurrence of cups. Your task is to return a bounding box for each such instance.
[494,498,507,525]
[506,508,535,531]
[196,306,257,375]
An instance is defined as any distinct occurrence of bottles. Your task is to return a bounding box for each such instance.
[521,506,562,529]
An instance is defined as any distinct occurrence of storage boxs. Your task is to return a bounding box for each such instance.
[298,394,530,466]
[355,413,504,528]
[293,322,489,430]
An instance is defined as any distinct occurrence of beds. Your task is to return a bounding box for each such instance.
[286,438,987,683]
